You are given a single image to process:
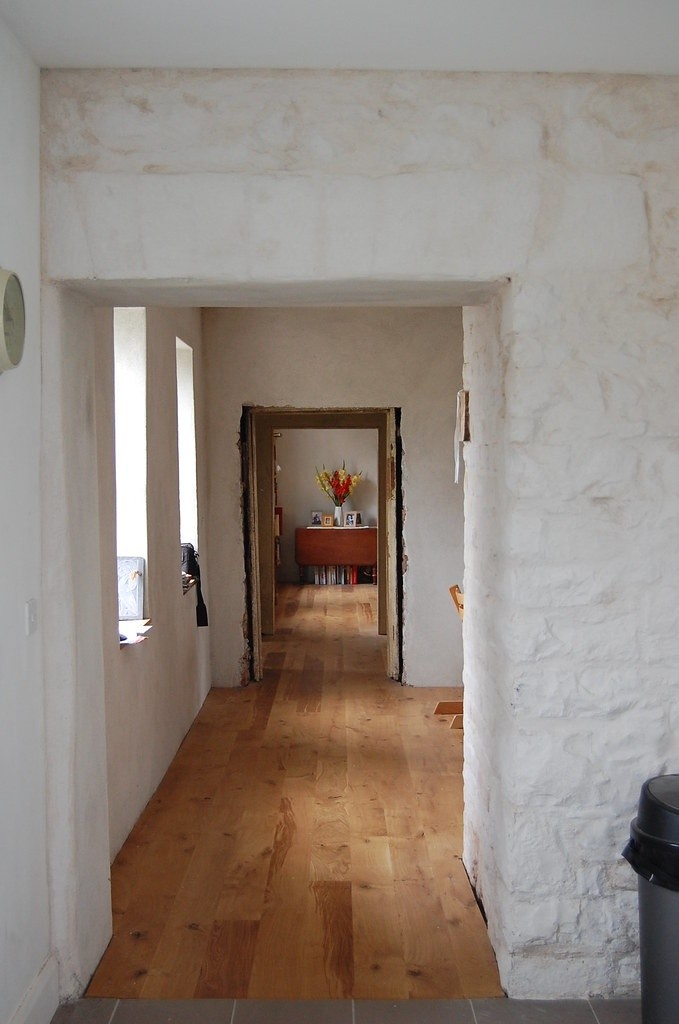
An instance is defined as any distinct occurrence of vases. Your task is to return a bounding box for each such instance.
[334,506,344,527]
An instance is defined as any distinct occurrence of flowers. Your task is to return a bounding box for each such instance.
[315,460,362,507]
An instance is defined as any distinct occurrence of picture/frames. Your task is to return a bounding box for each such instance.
[322,514,334,527]
[309,510,323,527]
[356,511,364,527]
[343,511,357,527]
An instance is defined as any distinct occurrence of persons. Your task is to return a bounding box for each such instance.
[346,514,354,525]
[314,514,320,524]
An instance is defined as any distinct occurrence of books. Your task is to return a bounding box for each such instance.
[313,565,357,585]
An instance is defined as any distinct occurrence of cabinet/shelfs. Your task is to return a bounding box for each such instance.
[295,527,377,585]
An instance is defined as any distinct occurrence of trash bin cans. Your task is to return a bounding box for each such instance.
[629,775,679,1024]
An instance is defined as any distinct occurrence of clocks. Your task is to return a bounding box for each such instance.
[0,266,26,376]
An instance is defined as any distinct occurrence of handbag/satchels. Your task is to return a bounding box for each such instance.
[181,543,197,576]
